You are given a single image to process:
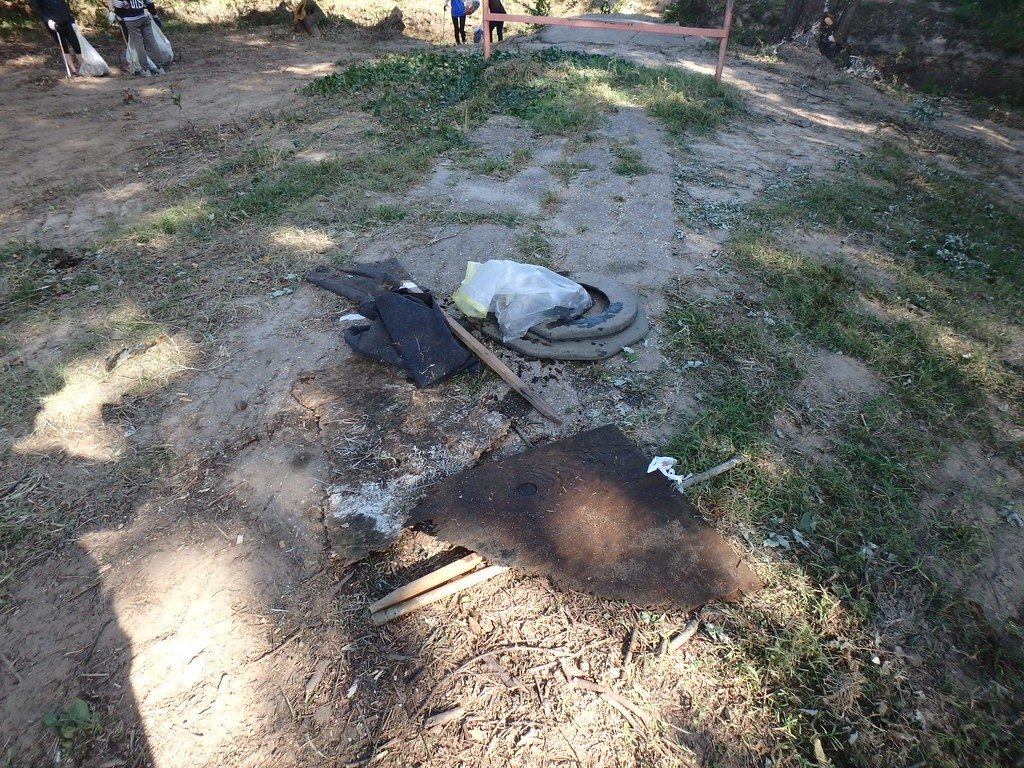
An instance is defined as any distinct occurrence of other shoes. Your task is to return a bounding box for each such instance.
[141,70,152,77]
[154,68,166,74]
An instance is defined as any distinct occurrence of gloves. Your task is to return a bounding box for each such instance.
[109,11,115,23]
[48,20,55,30]
[72,23,79,31]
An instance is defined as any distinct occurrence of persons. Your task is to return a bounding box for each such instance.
[107,0,166,77]
[37,0,81,75]
[443,1,468,45]
[489,0,506,43]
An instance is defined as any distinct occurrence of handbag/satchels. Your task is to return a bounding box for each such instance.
[474,23,484,43]
[465,0,480,15]
